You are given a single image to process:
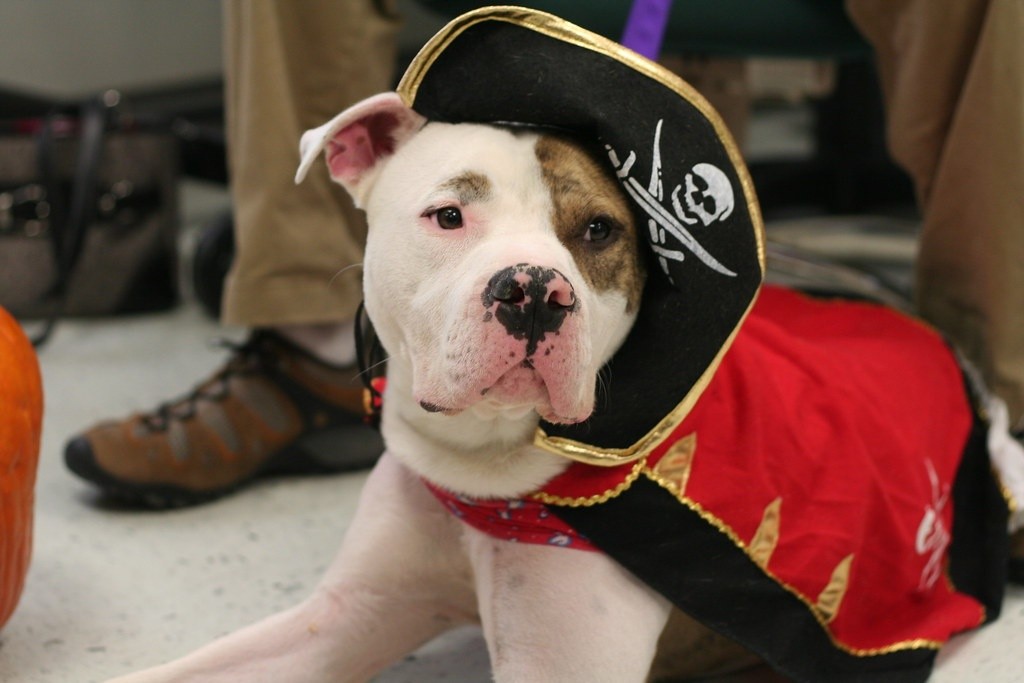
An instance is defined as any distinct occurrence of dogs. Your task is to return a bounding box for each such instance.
[114,92,1024,683]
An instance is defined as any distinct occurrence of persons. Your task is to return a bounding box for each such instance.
[64,0,1024,596]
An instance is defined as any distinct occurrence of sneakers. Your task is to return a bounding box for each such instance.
[65,330,387,494]
